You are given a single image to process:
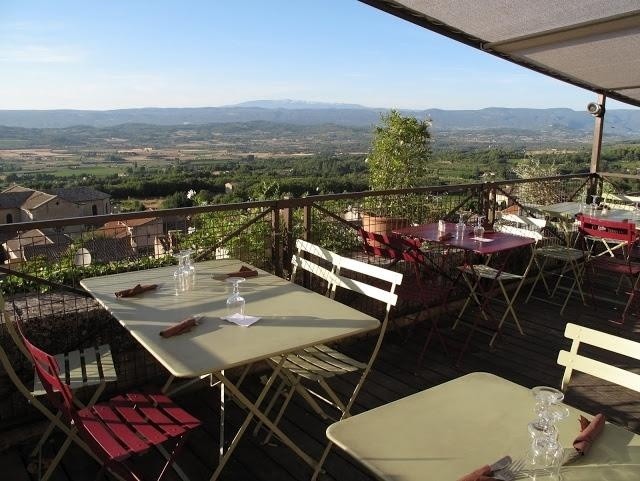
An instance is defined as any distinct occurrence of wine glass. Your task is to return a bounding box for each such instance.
[579,192,599,212]
[223,275,249,321]
[171,249,198,292]
[453,209,468,237]
[472,214,488,239]
[526,385,568,479]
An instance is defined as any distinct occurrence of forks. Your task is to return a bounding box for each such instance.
[490,454,525,481]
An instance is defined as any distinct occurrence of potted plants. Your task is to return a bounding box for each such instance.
[358,110,435,260]
